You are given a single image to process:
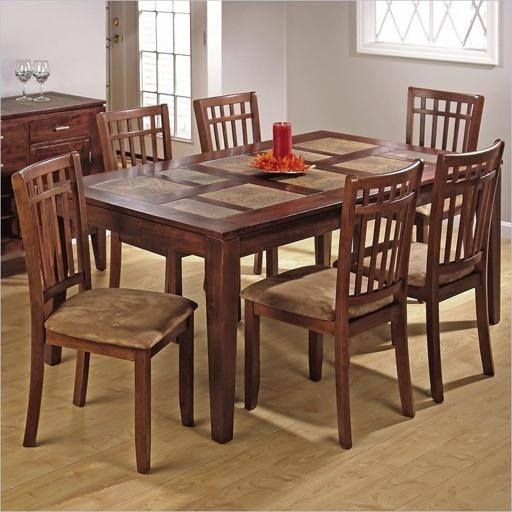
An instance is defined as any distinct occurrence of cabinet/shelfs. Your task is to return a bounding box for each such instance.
[1,91,108,281]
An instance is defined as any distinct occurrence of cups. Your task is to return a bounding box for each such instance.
[273,121,292,162]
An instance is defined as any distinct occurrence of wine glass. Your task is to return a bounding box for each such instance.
[14,60,33,102]
[32,60,51,102]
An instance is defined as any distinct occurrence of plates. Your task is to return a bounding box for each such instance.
[248,161,317,174]
